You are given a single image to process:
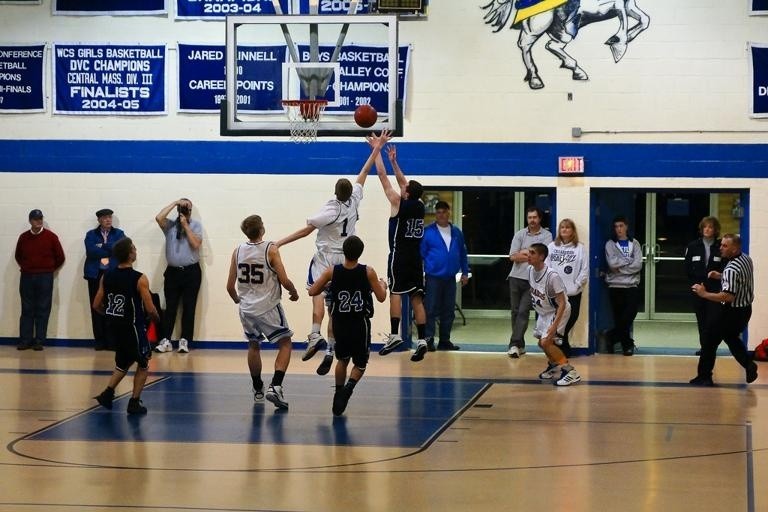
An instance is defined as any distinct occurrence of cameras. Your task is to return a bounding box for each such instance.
[177,205,190,216]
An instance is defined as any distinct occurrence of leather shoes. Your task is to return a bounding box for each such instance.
[33,343,42,350]
[745,361,758,383]
[95,342,105,350]
[107,343,115,350]
[690,376,713,386]
[17,344,31,349]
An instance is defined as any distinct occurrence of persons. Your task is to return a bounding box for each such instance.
[690,234,758,387]
[306,235,387,421]
[545,217,588,354]
[90,237,160,420]
[155,194,203,356]
[420,199,469,352]
[226,212,298,412]
[504,208,552,359]
[81,209,126,352]
[684,215,730,355]
[363,129,428,362]
[602,214,642,355]
[526,242,581,389]
[272,127,394,375]
[12,209,65,353]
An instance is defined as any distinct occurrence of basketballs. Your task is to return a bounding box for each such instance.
[355,105,377,127]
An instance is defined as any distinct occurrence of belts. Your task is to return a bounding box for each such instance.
[167,265,192,270]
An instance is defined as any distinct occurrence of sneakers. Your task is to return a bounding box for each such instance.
[265,383,289,411]
[300,330,353,416]
[94,387,114,411]
[624,340,635,355]
[507,345,521,358]
[154,338,173,353]
[378,333,459,362]
[127,396,147,416]
[600,328,615,354]
[252,381,265,403]
[177,339,189,353]
[520,347,525,355]
[553,368,581,386]
[540,361,569,378]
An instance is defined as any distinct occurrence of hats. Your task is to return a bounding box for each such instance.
[435,200,450,210]
[95,208,113,217]
[29,210,43,219]
